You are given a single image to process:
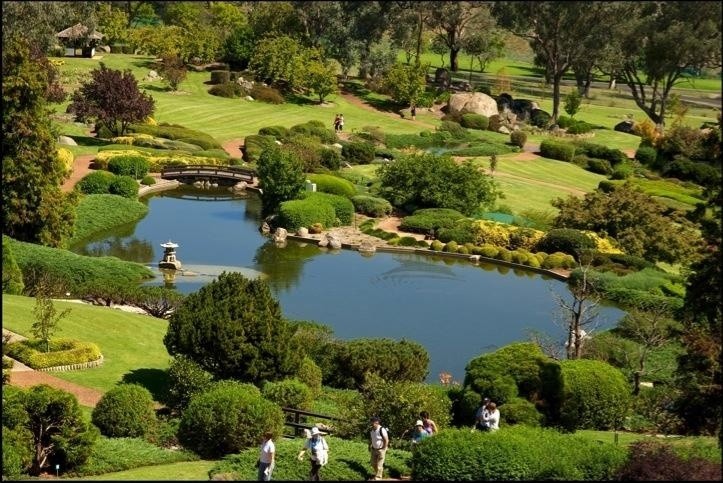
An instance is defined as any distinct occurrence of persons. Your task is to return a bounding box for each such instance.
[470,396,490,432]
[409,419,428,451]
[332,113,339,131]
[480,401,500,431]
[296,426,329,481]
[367,415,388,480]
[410,101,416,120]
[338,113,343,131]
[419,410,437,436]
[256,429,275,482]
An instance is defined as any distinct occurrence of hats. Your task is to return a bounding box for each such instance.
[414,420,425,428]
[310,427,322,436]
